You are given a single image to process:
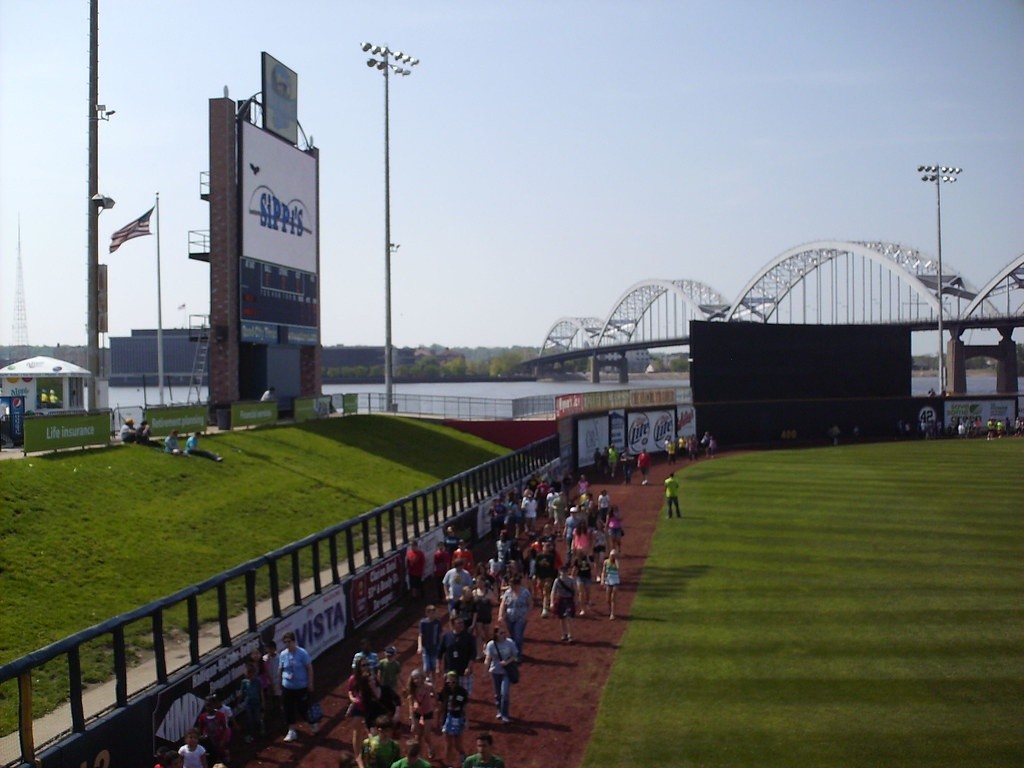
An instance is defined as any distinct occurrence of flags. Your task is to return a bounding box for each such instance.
[110,206,153,253]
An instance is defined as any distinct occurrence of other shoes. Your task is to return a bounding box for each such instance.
[540,611,548,618]
[561,635,565,640]
[580,608,585,615]
[568,634,574,643]
[495,712,502,719]
[609,615,616,621]
[312,723,320,733]
[501,716,509,723]
[428,746,436,757]
[283,729,298,742]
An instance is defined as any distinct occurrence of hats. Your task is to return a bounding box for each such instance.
[569,506,578,515]
[384,647,396,655]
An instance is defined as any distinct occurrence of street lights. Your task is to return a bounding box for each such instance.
[918,164,963,396]
[357,41,420,412]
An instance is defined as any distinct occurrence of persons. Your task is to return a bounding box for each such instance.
[947,416,982,432]
[121,419,224,462]
[260,387,275,402]
[594,444,650,485]
[831,419,942,446]
[41,388,60,403]
[664,432,715,464]
[664,472,679,518]
[958,417,1024,440]
[406,474,623,658]
[153,606,519,767]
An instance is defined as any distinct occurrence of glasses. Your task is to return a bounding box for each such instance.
[359,663,369,667]
[446,680,457,685]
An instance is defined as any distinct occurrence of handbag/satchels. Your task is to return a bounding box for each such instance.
[305,693,323,725]
[505,657,519,684]
